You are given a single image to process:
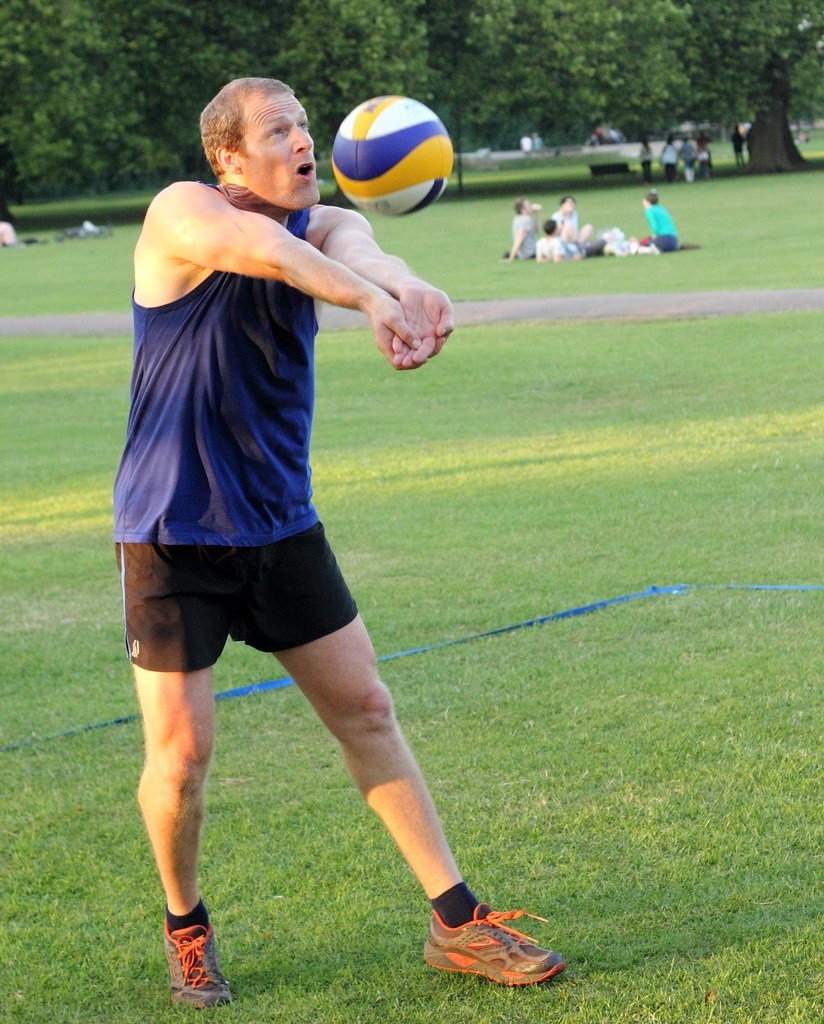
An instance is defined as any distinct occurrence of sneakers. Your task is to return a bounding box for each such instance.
[162,916,231,1008]
[423,901,567,985]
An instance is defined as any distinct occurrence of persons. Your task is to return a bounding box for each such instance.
[731,124,746,167]
[112,77,565,1010]
[643,192,679,252]
[551,194,592,248]
[0,203,22,248]
[519,132,544,152]
[639,136,654,183]
[535,221,615,263]
[500,198,542,263]
[659,130,713,184]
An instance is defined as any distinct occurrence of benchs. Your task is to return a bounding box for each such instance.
[591,164,629,178]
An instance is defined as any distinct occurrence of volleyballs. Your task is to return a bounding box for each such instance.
[331,94,455,219]
